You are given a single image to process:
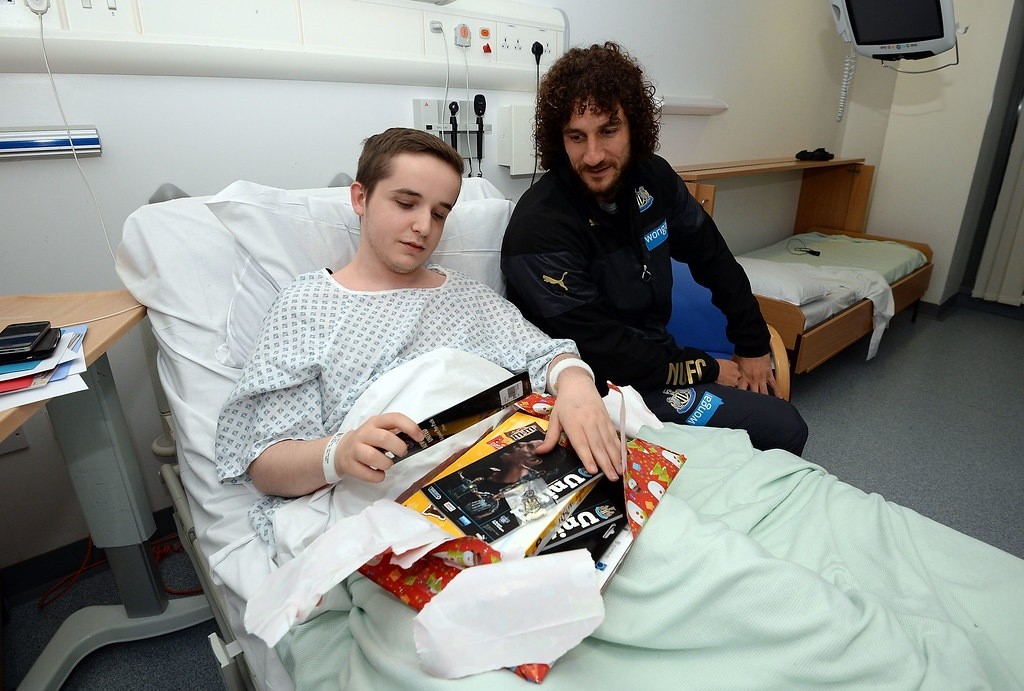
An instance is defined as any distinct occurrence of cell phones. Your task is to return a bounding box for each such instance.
[0,320,50,356]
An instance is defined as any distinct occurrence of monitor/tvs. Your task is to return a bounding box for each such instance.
[828,0,956,61]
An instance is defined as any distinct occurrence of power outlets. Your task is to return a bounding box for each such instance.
[497,21,556,67]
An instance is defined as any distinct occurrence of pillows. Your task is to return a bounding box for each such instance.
[736,256,835,307]
[203,179,515,370]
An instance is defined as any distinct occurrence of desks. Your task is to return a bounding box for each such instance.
[1,288,214,691]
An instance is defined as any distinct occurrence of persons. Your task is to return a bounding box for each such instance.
[450,439,567,520]
[215,127,981,686]
[501,41,809,461]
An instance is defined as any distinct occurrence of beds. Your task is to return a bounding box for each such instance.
[115,174,1022,691]
[735,225,933,375]
